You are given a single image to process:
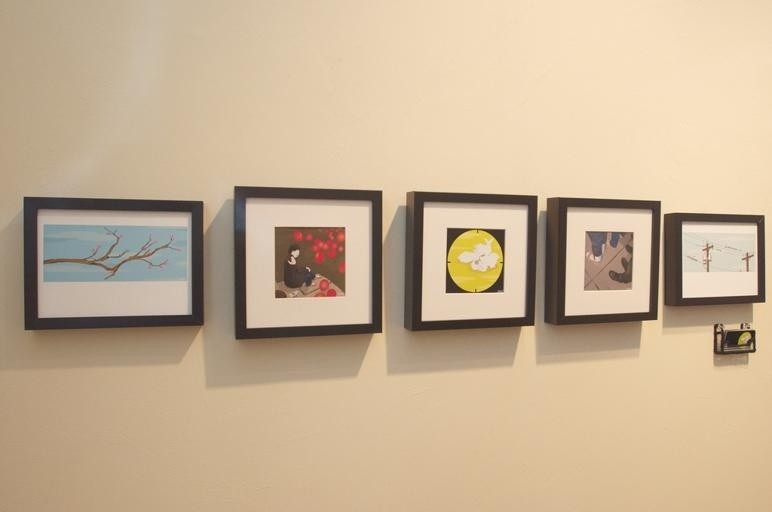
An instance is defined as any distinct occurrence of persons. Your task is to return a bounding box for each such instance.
[610,232,627,249]
[623,232,633,253]
[609,255,632,284]
[283,243,321,289]
[584,232,608,264]
